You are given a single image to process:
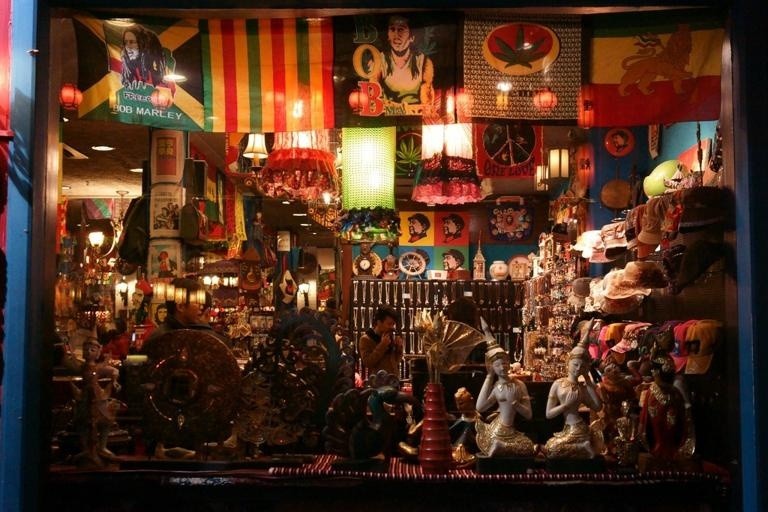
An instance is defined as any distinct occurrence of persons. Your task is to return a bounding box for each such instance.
[408,213,466,279]
[64,340,121,456]
[545,347,603,459]
[359,306,403,381]
[474,347,534,459]
[138,278,231,355]
[367,14,434,105]
[121,26,166,87]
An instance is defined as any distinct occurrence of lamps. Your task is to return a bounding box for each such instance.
[534,144,570,194]
[242,122,486,244]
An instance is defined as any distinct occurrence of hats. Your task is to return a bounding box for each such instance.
[408,213,430,230]
[568,189,723,374]
[442,213,464,229]
[442,249,465,264]
